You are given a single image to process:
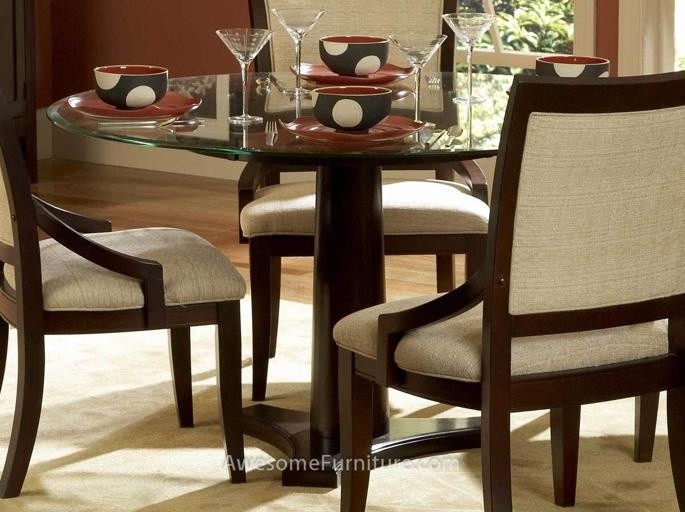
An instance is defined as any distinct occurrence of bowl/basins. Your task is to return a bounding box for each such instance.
[319,35,389,78]
[535,55,611,81]
[311,84,390,134]
[94,65,168,109]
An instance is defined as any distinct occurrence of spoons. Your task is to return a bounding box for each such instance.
[445,126,463,147]
[93,114,178,129]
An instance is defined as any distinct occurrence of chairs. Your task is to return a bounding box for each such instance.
[0,89,246,499]
[239,0,489,401]
[333,70,685,512]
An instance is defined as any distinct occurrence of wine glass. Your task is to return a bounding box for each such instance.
[216,28,276,125]
[227,121,260,150]
[270,7,324,130]
[388,33,447,128]
[442,13,496,103]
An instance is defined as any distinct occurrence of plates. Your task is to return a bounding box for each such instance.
[286,115,426,145]
[288,62,414,84]
[69,94,205,120]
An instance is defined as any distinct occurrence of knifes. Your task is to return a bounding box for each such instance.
[423,71,432,85]
[268,71,288,90]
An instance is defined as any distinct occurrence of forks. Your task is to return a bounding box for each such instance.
[265,120,278,147]
